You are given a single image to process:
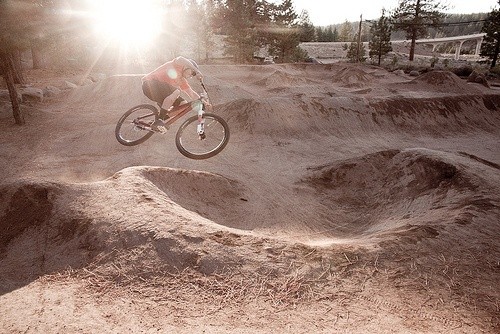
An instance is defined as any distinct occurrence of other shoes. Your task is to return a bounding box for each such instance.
[150,120,167,134]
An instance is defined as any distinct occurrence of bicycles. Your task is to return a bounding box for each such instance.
[114,71,230,160]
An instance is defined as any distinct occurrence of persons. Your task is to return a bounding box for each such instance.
[142,55,211,135]
